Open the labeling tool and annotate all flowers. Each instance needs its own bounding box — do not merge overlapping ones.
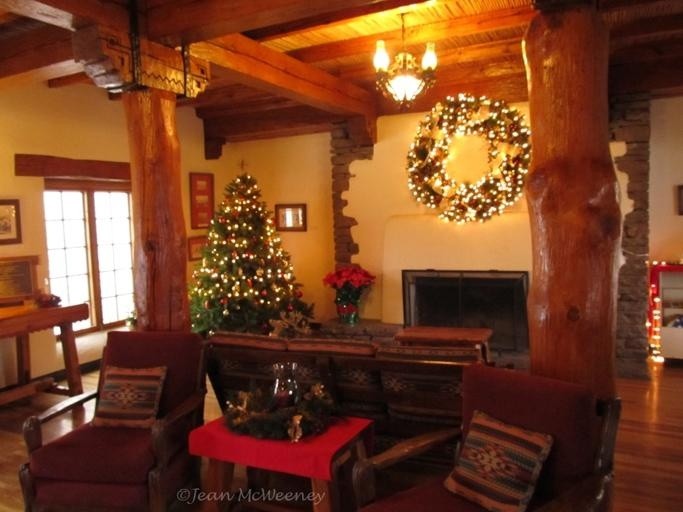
[323,262,376,304]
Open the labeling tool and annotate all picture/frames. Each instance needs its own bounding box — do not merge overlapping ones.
[190,236,207,260]
[0,198,23,244]
[0,255,38,303]
[275,204,306,232]
[190,173,214,231]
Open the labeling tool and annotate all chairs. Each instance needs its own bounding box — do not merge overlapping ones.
[287,331,383,428]
[208,328,288,414]
[376,344,491,424]
[364,364,621,511]
[19,330,205,511]
[372,12,437,109]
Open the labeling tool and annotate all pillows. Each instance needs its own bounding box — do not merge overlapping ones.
[447,410,553,512]
[93,366,168,429]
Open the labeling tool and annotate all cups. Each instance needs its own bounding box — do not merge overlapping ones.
[270,361,300,408]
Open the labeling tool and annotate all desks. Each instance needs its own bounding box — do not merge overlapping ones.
[310,319,404,341]
[1,304,89,408]
[187,416,377,511]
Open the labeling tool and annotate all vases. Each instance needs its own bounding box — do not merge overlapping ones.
[340,302,359,321]
